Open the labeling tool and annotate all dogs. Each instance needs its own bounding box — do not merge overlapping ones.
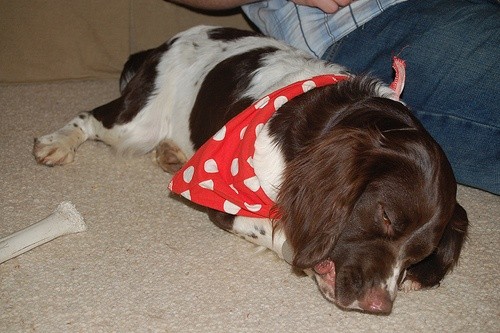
[32,23,469,316]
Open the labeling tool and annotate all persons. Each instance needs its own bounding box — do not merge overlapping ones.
[170,0,500,196]
